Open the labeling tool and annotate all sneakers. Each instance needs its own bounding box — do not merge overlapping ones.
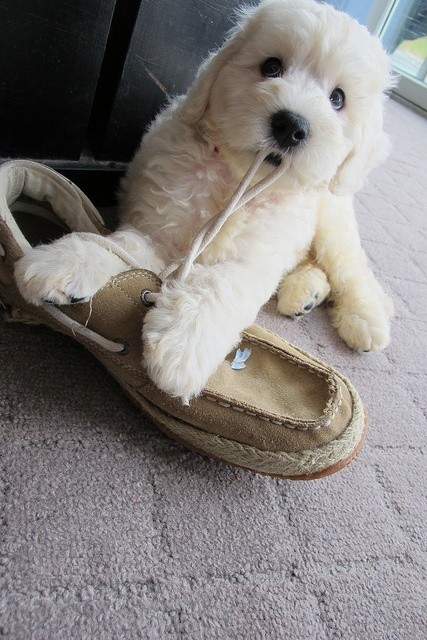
[1,159,368,480]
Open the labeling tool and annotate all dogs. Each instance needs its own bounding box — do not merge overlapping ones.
[12,0,396,398]
